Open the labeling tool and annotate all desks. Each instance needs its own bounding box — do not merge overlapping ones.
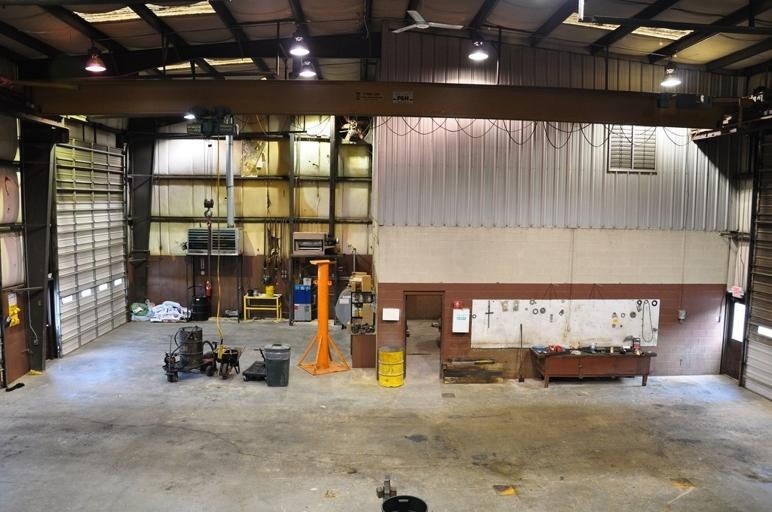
[529,345,656,387]
[289,256,339,327]
[242,291,282,320]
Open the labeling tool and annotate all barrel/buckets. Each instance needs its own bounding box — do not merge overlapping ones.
[180,326,202,367]
[191,295,210,321]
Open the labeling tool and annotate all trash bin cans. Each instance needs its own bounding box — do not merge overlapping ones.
[264,343,291,385]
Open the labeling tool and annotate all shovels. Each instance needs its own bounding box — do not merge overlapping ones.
[520,324,523,382]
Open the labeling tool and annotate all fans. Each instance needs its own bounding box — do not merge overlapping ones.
[390,9,464,36]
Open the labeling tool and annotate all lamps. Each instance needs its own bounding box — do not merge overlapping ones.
[83,34,107,72]
[658,54,683,89]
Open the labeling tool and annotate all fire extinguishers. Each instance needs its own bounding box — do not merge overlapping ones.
[205,281,212,298]
